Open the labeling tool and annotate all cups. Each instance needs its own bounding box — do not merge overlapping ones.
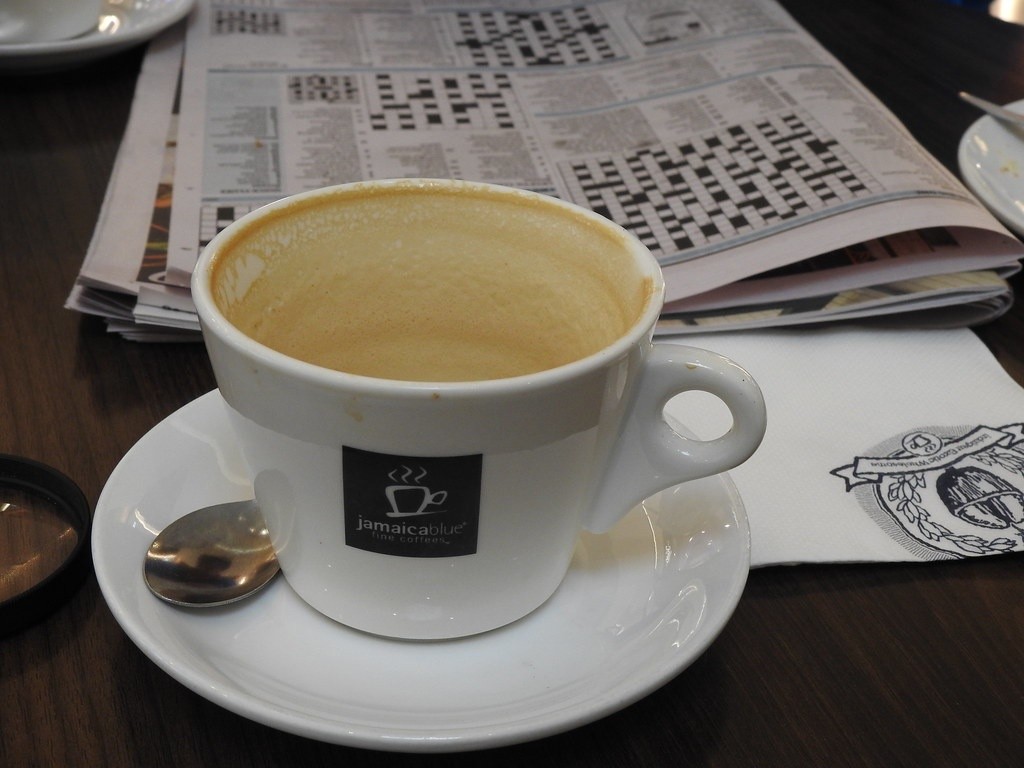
[191,179,766,643]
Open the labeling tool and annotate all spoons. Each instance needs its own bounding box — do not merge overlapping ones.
[144,499,277,610]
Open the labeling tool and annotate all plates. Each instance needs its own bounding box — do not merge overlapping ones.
[90,386,753,752]
[956,96,1024,236]
[0,0,195,56]
